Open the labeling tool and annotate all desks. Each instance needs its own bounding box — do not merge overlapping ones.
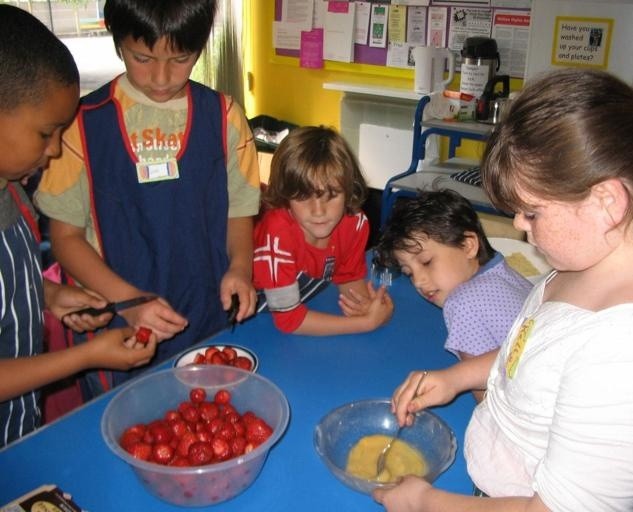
[1,238,559,512]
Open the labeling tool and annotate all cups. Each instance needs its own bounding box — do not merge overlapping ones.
[486,97,512,126]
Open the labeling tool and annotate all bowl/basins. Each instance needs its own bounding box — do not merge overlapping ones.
[170,342,260,398]
[98,364,290,510]
[315,395,459,499]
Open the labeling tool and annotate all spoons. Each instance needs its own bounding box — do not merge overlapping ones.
[372,371,428,476]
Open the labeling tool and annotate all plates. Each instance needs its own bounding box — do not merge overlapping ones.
[484,234,552,287]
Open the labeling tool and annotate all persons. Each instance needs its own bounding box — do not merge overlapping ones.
[371,175,538,406]
[371,66,632,511]
[0,0,158,449]
[33,0,263,408]
[252,125,394,338]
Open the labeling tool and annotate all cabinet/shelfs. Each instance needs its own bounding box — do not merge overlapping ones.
[377,90,530,234]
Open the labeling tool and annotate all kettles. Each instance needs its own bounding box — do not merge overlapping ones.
[409,44,454,96]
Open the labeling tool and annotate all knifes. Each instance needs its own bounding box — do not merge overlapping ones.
[59,292,159,324]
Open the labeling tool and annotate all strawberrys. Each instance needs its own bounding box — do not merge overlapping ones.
[179,345,252,386]
[135,326,152,344]
[117,388,274,508]
